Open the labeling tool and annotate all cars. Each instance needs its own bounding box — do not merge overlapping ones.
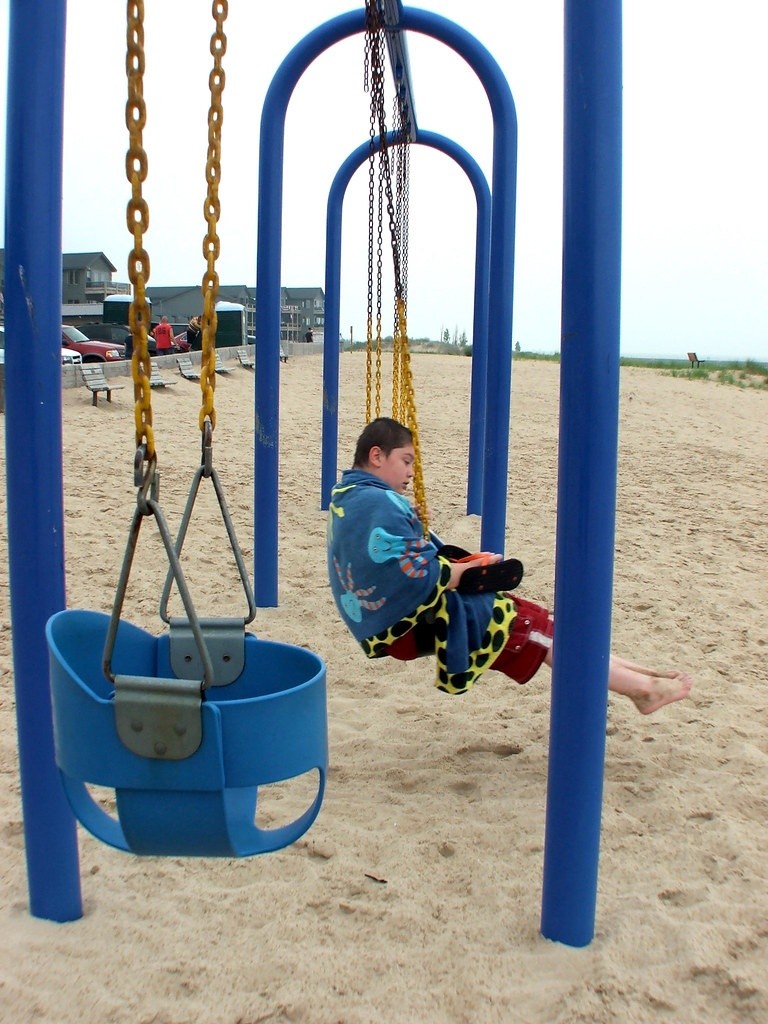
[0,326,84,366]
[61,324,126,363]
[171,331,192,353]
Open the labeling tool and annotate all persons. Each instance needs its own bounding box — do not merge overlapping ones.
[327,417,693,715]
[187,314,202,348]
[305,328,313,343]
[124,329,133,360]
[150,316,180,355]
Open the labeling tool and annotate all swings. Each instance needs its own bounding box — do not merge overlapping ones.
[364,0,433,663]
[44,0,329,860]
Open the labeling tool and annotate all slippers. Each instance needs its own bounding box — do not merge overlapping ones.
[459,556,523,593]
[435,545,496,563]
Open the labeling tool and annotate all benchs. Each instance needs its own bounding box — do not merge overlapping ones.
[280,345,294,363]
[140,362,178,389]
[79,364,125,407]
[214,354,237,374]
[688,352,705,368]
[236,349,254,368]
[176,358,201,379]
